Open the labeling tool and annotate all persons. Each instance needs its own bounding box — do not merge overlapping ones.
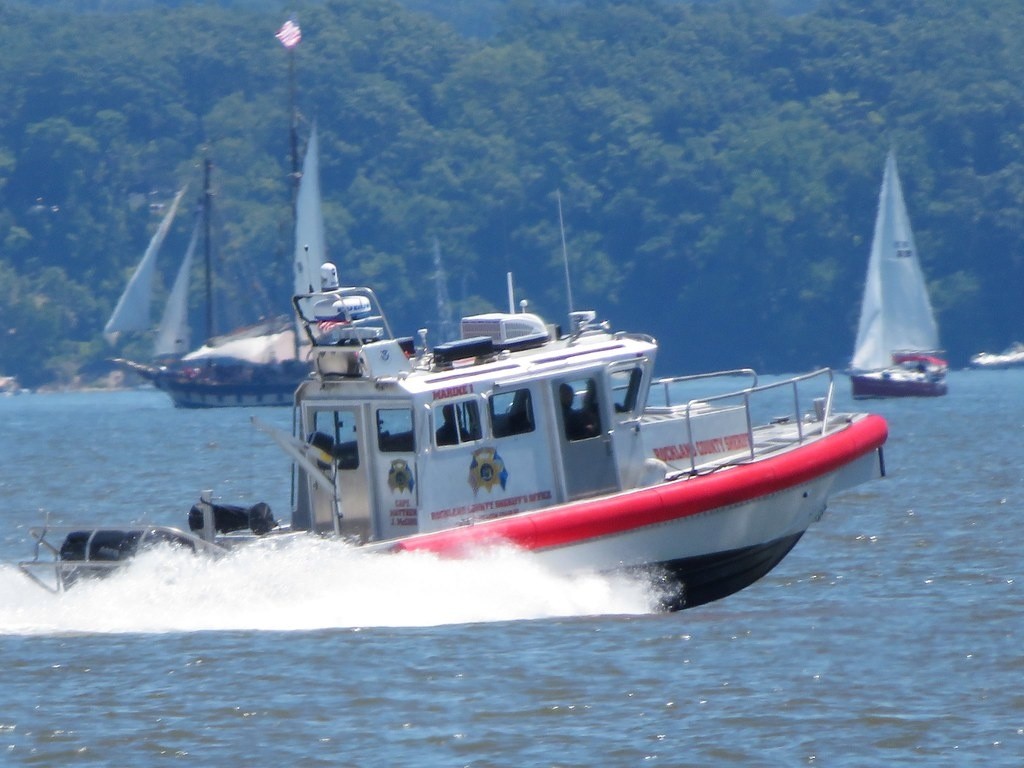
[560,383,598,440]
[916,361,925,371]
[435,404,470,446]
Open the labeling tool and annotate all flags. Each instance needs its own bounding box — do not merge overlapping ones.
[275,11,302,50]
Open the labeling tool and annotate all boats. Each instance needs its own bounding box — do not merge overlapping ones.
[2,187,886,622]
[968,341,1024,373]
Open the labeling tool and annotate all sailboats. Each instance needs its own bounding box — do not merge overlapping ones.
[99,16,417,410]
[844,155,948,402]
